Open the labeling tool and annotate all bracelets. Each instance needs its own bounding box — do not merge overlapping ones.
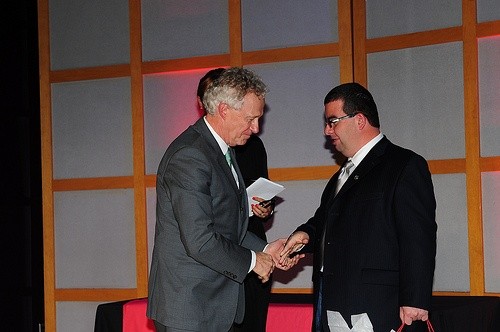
[270,209,273,216]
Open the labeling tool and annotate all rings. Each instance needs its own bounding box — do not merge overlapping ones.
[259,212,263,215]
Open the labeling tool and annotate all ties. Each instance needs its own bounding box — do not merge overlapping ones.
[334,159,354,192]
[225,150,239,181]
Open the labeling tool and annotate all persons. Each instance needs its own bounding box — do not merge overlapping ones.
[197,67,273,332]
[279,82,438,332]
[146,67,305,332]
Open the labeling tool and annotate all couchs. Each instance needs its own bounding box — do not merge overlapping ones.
[93,293,500,332]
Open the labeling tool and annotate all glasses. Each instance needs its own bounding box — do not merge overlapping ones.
[326,112,356,126]
